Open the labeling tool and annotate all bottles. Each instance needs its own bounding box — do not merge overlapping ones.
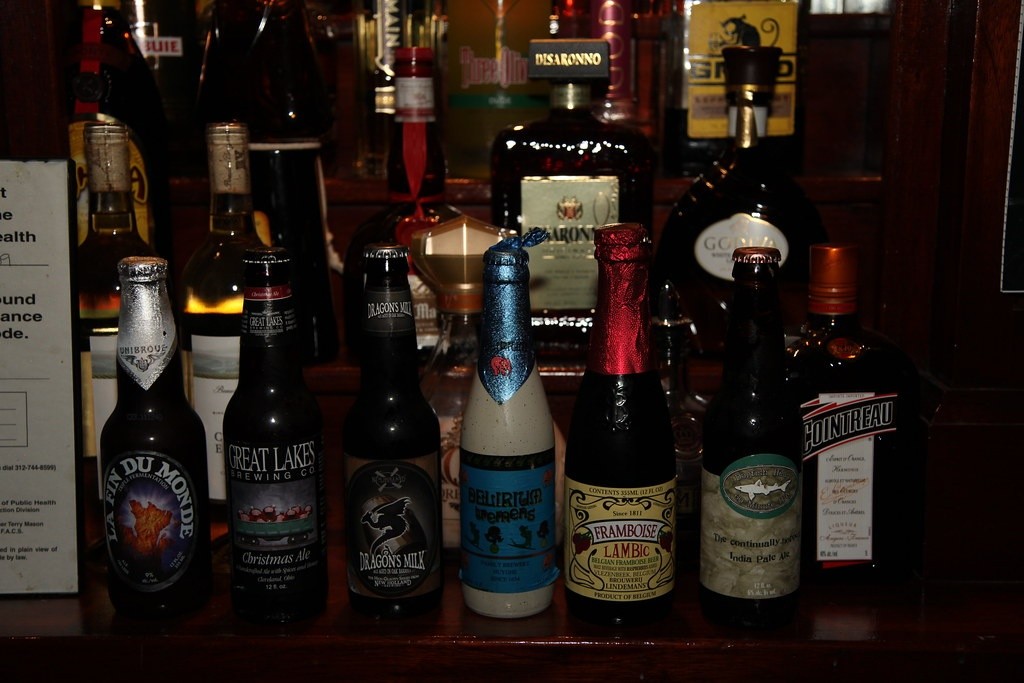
[701,248,809,624]
[222,243,326,632]
[71,121,159,549]
[656,43,827,358]
[342,247,443,613]
[101,255,212,622]
[636,281,709,575]
[414,217,516,552]
[248,141,334,366]
[490,41,654,356]
[341,44,467,361]
[561,225,682,623]
[178,121,257,523]
[461,248,557,618]
[787,242,918,588]
[63,0,809,186]
